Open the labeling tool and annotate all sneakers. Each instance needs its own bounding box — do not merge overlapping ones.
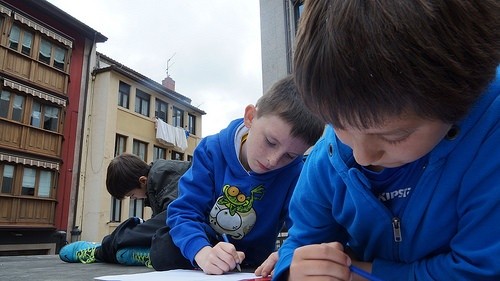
[116,246,154,269]
[59,241,104,263]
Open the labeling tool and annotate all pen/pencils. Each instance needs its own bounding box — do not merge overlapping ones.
[221,233,243,273]
[347,264,383,281]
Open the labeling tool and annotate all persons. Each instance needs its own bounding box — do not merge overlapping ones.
[59,74,325,278]
[106,153,192,218]
[273,1,500,281]
